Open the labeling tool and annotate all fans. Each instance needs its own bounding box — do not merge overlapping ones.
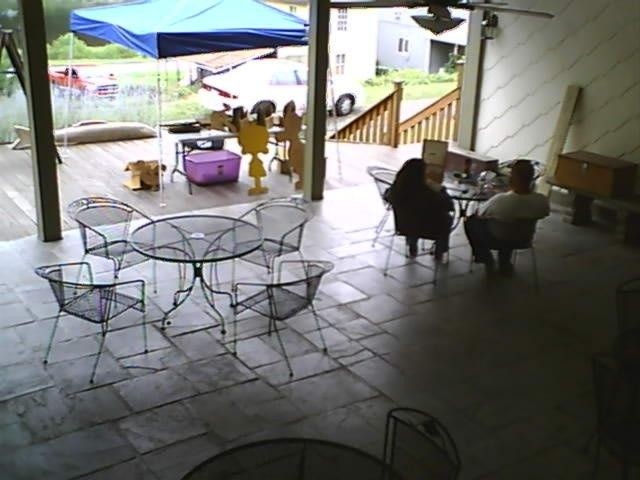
[324,0,558,24]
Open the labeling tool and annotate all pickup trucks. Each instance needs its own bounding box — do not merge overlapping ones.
[49,63,119,101]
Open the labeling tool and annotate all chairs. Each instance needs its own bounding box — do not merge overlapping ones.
[227,257,334,382]
[365,158,556,289]
[34,261,153,387]
[578,275,639,480]
[375,402,464,480]
[63,194,159,302]
[224,194,319,291]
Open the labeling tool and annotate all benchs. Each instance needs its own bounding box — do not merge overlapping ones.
[545,173,615,231]
[590,192,639,243]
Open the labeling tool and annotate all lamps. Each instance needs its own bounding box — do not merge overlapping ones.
[479,11,503,42]
[408,6,465,35]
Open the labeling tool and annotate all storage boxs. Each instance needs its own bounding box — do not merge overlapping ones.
[555,149,638,202]
[183,145,243,187]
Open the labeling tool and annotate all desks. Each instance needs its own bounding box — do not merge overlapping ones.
[165,122,307,197]
[122,215,267,336]
[174,433,407,478]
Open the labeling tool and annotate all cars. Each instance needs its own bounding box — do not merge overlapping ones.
[198,57,365,120]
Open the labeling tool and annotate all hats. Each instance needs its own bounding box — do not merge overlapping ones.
[507,160,535,183]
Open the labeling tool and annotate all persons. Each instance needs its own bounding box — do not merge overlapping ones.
[383,156,453,255]
[463,161,550,270]
